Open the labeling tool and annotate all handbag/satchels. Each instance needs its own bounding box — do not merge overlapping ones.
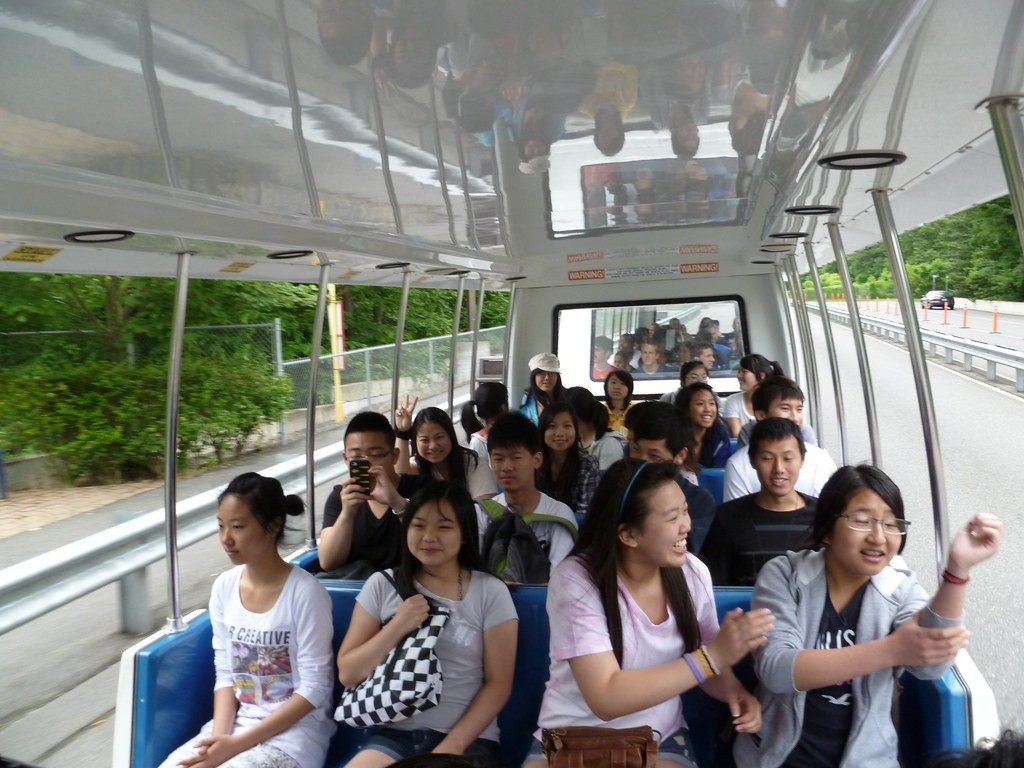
[334,571,451,729]
[538,726,661,768]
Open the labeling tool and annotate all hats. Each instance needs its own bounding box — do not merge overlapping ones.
[528,352,561,374]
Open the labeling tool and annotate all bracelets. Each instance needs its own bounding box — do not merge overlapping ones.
[395,425,412,440]
[697,645,720,677]
[391,498,410,517]
[683,654,704,686]
[943,571,968,584]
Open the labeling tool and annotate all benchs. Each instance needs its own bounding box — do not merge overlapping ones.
[113,438,999,767]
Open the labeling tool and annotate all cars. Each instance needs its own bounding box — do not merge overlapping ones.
[921,291,954,310]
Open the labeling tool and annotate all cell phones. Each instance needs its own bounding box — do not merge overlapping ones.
[350,460,370,497]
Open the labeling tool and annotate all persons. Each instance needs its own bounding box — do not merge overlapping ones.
[395,394,498,502]
[319,411,428,578]
[701,417,818,586]
[521,352,697,524]
[461,383,508,454]
[658,354,839,502]
[633,401,715,553]
[160,473,336,768]
[520,458,774,768]
[731,463,1001,768]
[474,412,579,585]
[316,0,793,224]
[336,479,519,768]
[594,318,742,378]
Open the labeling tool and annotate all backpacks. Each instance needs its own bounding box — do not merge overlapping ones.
[471,497,578,586]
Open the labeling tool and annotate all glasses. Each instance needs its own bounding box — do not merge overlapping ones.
[343,451,392,466]
[833,512,911,535]
